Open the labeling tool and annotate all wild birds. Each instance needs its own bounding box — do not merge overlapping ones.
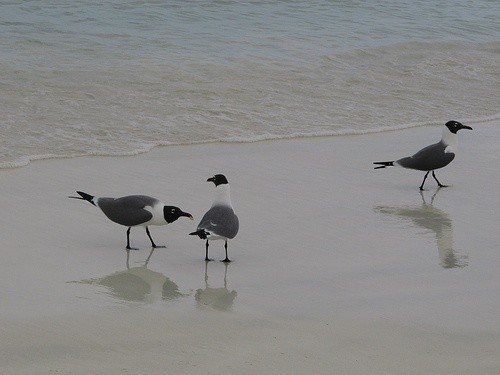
[188,173,239,262]
[65,247,193,311]
[373,120,473,190]
[67,188,194,251]
[373,187,470,268]
[194,261,238,312]
